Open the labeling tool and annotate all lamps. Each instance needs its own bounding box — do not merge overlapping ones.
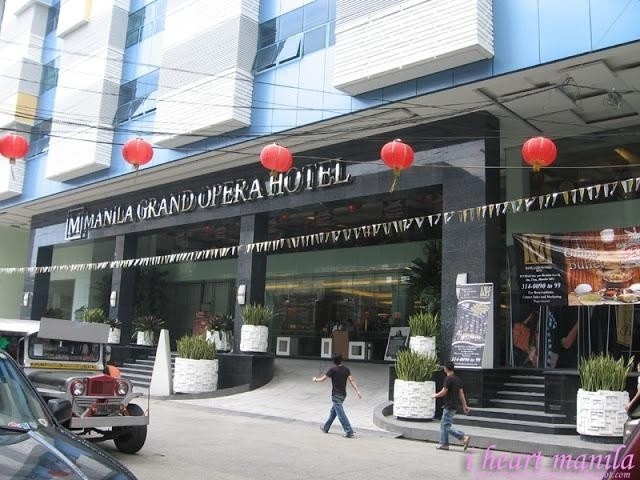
[456,273,469,301]
[23,292,30,307]
[110,290,117,308]
[237,283,247,306]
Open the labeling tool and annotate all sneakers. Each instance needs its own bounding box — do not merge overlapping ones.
[320,426,354,437]
[436,436,471,451]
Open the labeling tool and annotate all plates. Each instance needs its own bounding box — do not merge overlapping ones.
[578,293,604,306]
[598,287,621,299]
[602,270,633,283]
[616,293,640,305]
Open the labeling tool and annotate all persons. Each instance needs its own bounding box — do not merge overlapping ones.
[430,360,470,451]
[330,319,344,336]
[344,319,356,341]
[312,351,362,440]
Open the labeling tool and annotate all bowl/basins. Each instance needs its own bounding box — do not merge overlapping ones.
[574,288,592,297]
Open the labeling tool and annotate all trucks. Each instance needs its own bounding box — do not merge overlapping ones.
[0,316,148,454]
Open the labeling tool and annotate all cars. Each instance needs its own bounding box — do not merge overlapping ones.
[0,349,140,480]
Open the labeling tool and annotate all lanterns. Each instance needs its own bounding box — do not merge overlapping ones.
[122,137,153,168]
[521,136,558,170]
[380,136,413,177]
[260,143,292,177]
[0,132,29,164]
